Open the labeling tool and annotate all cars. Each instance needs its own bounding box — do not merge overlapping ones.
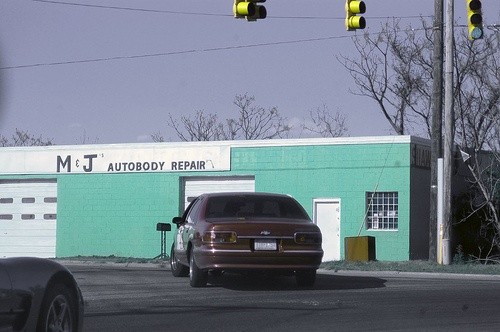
[170,192,324,287]
[0,258,84,331]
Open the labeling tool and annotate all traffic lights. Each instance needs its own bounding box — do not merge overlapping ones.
[466,0,483,40]
[233,0,269,23]
[345,0,366,31]
